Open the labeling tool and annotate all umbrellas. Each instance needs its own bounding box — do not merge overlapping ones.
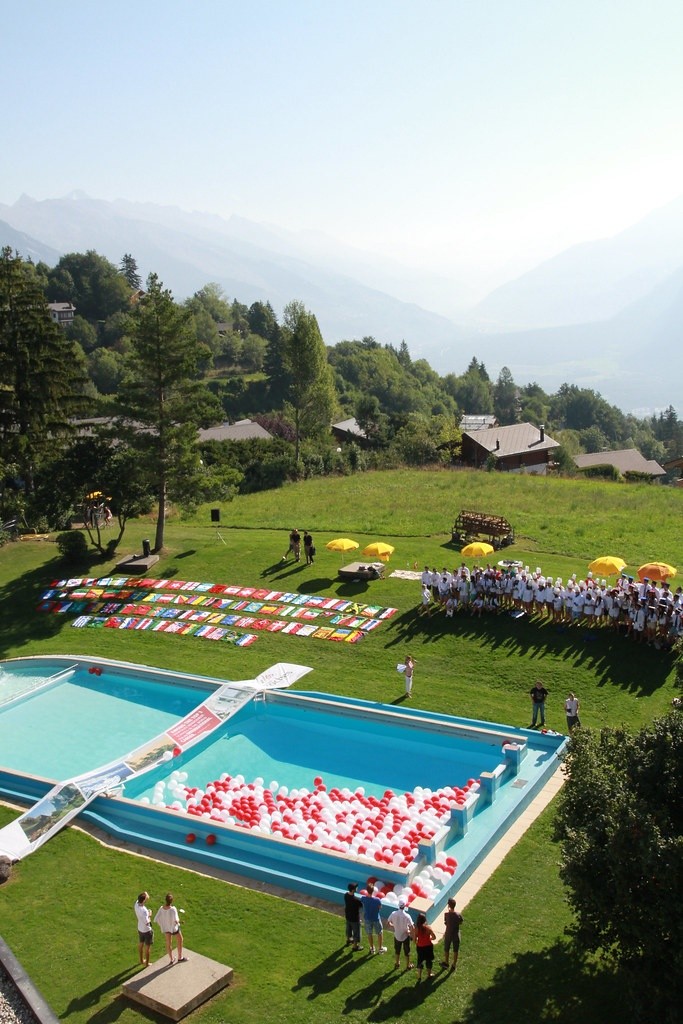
[588,556,627,585]
[461,542,494,567]
[637,562,677,584]
[326,538,395,563]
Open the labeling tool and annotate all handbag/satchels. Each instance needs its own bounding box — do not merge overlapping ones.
[310,548,317,555]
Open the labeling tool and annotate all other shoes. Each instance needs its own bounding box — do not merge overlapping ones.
[407,963,415,970]
[379,946,387,954]
[352,945,364,951]
[346,939,354,944]
[439,961,449,968]
[395,960,400,968]
[427,974,435,978]
[178,958,188,963]
[451,963,457,970]
[169,957,177,964]
[370,946,376,954]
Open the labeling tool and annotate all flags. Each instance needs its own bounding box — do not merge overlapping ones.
[36,578,398,647]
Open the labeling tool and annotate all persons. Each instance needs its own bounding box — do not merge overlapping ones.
[153,893,190,964]
[361,883,387,954]
[529,682,548,727]
[85,502,113,528]
[421,561,683,650]
[404,656,415,698]
[344,883,364,951]
[440,898,463,971]
[413,913,436,980]
[134,891,155,967]
[387,901,414,970]
[283,529,315,565]
[564,693,581,731]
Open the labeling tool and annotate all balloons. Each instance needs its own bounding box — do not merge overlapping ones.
[142,740,523,907]
[88,667,101,676]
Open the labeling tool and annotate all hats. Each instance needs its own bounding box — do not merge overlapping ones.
[507,561,606,596]
[347,883,359,891]
[607,574,683,613]
[399,899,407,908]
